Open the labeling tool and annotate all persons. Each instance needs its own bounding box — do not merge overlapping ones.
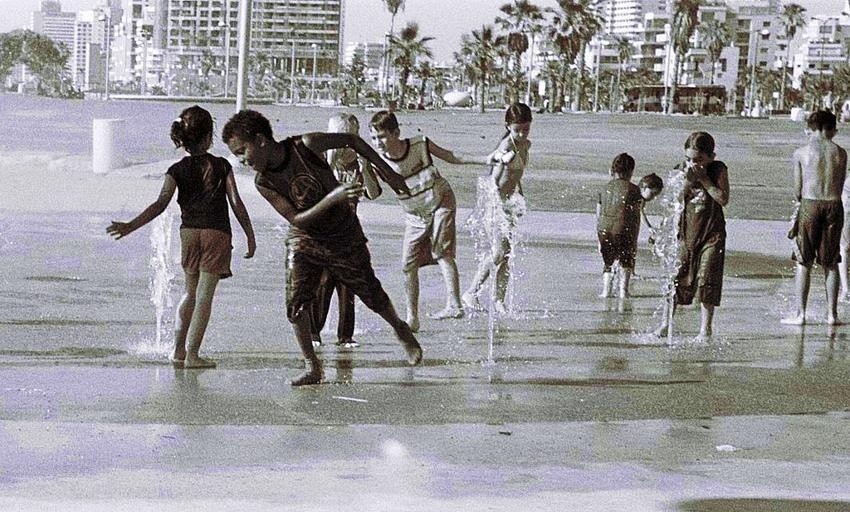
[780,110,847,325]
[221,108,424,389]
[595,152,640,304]
[649,131,731,343]
[610,170,664,279]
[839,176,850,303]
[368,113,501,334]
[103,105,256,368]
[310,112,384,352]
[460,101,534,320]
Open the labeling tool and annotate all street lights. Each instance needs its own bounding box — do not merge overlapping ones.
[136,28,148,95]
[748,28,770,115]
[218,21,231,98]
[811,15,840,111]
[310,43,317,105]
[97,7,111,101]
